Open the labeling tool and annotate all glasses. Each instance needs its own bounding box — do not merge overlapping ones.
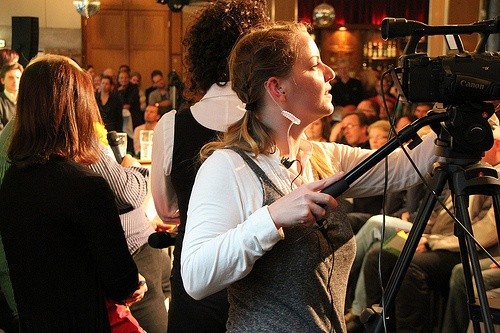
[368,134,388,139]
[346,123,360,129]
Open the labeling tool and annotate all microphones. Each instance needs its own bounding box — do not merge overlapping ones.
[281,109,301,125]
[106,131,123,164]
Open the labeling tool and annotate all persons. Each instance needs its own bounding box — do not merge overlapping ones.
[180,21,446,333]
[304,62,500,333]
[151,0,307,333]
[0,49,173,332]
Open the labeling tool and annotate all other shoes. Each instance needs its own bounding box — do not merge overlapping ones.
[344,308,365,333]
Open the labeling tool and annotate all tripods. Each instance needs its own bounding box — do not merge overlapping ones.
[314,103,500,333]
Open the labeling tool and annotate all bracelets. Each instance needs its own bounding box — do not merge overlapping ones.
[424,243,432,251]
